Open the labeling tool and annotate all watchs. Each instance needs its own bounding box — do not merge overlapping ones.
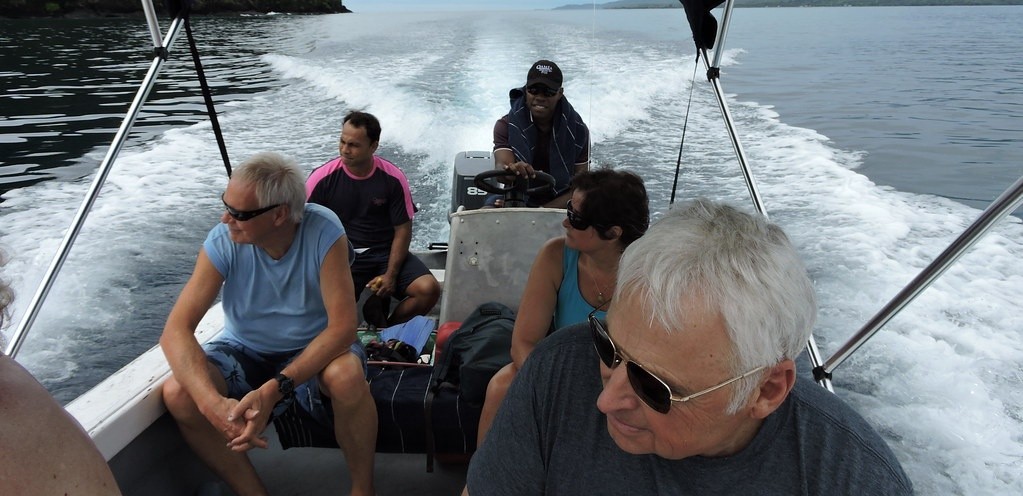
[275,374,294,395]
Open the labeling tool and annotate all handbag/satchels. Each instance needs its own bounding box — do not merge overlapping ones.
[432,301,515,403]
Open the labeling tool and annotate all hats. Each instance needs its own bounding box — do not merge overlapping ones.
[527,60,563,91]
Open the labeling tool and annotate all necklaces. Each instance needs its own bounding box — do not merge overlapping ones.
[591,274,610,303]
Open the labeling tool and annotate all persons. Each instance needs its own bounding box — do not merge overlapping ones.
[463,197,908,495]
[482,60,592,208]
[160,153,378,495]
[304,111,441,324]
[0,242,121,496]
[477,165,652,450]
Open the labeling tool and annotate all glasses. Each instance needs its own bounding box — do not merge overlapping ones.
[222,192,280,221]
[566,199,625,232]
[587,296,767,415]
[526,84,561,97]
